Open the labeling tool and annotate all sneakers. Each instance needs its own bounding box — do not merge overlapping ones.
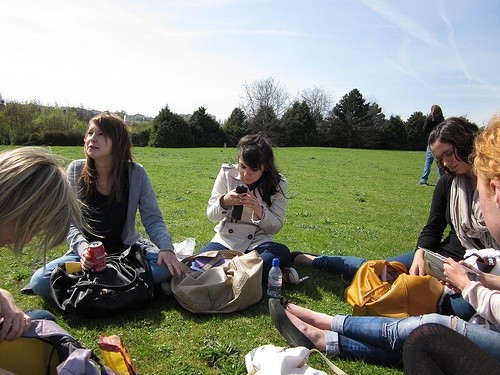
[282,267,299,286]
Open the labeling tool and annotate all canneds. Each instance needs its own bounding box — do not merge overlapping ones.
[88,241,107,272]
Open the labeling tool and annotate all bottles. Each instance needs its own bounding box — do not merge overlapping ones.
[267,258,283,298]
[467,309,490,332]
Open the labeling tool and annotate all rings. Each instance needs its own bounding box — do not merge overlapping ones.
[167,264,171,266]
[240,200,242,203]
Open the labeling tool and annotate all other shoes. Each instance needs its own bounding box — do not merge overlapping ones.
[160,282,171,296]
[277,296,289,309]
[290,251,321,266]
[269,298,316,351]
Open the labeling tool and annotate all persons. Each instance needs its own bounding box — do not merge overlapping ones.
[418,105,445,185]
[200,134,298,287]
[0,146,104,375]
[269,117,500,375]
[30,111,183,325]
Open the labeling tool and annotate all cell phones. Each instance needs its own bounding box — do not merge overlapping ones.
[236,186,247,196]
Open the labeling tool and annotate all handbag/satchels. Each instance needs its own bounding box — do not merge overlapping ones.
[172,251,265,315]
[344,259,444,319]
[50,244,157,323]
[0,319,118,375]
[243,343,347,375]
